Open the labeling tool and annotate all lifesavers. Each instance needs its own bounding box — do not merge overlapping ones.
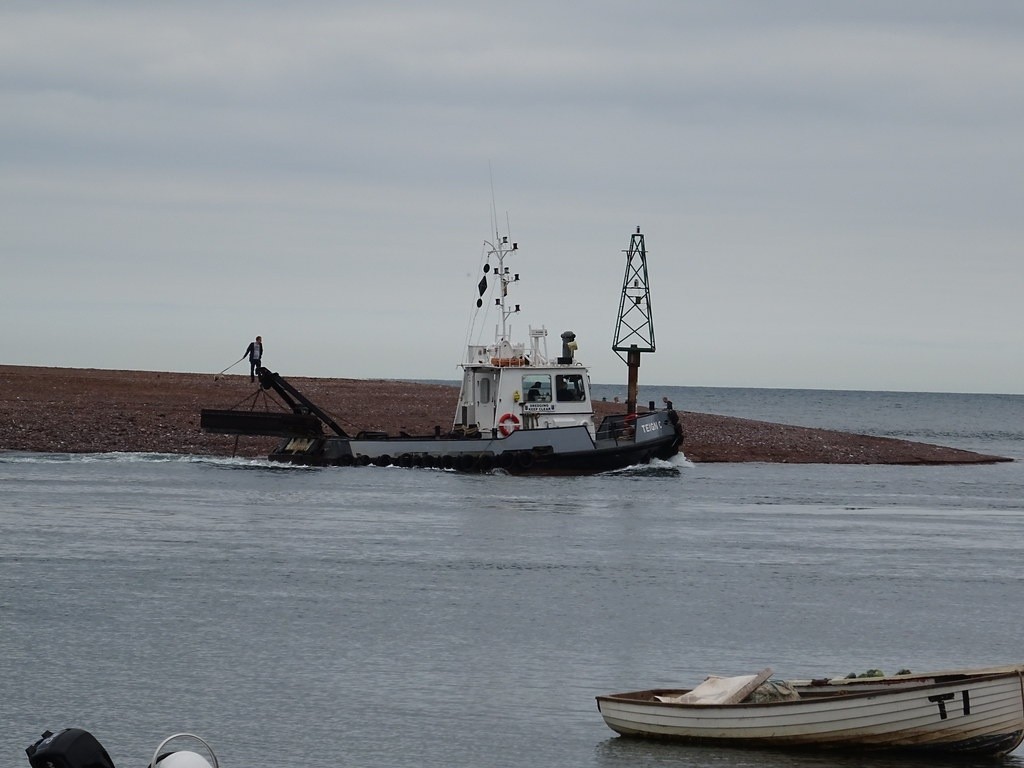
[624,414,639,434]
[499,414,519,436]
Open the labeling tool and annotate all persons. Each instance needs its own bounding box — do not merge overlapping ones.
[528,382,541,401]
[243,336,263,383]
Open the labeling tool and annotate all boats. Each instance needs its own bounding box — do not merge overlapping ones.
[347,164,682,474]
[594,666,1023,765]
